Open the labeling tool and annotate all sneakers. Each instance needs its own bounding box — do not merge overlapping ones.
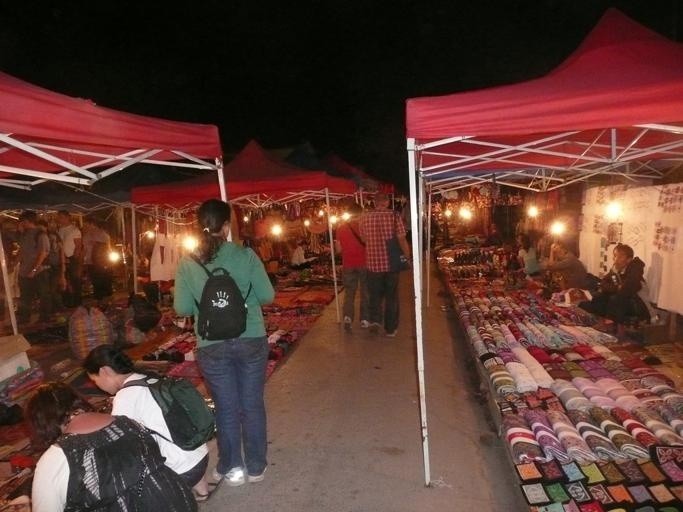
[344,317,398,337]
[211,467,267,487]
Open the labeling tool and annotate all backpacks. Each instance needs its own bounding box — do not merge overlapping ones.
[119,370,215,451]
[190,253,252,341]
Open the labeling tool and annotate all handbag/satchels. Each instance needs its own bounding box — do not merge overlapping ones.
[387,237,410,273]
[68,307,115,361]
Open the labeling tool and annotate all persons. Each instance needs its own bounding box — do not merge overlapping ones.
[86,343,216,502]
[288,240,319,271]
[171,198,275,487]
[429,206,590,291]
[588,242,655,324]
[4,208,115,310]
[398,192,412,255]
[19,377,198,512]
[333,201,372,334]
[357,193,412,339]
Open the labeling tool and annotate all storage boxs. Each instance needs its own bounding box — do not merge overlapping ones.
[0,334,33,382]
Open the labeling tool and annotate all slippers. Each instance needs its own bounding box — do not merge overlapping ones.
[196,477,224,504]
